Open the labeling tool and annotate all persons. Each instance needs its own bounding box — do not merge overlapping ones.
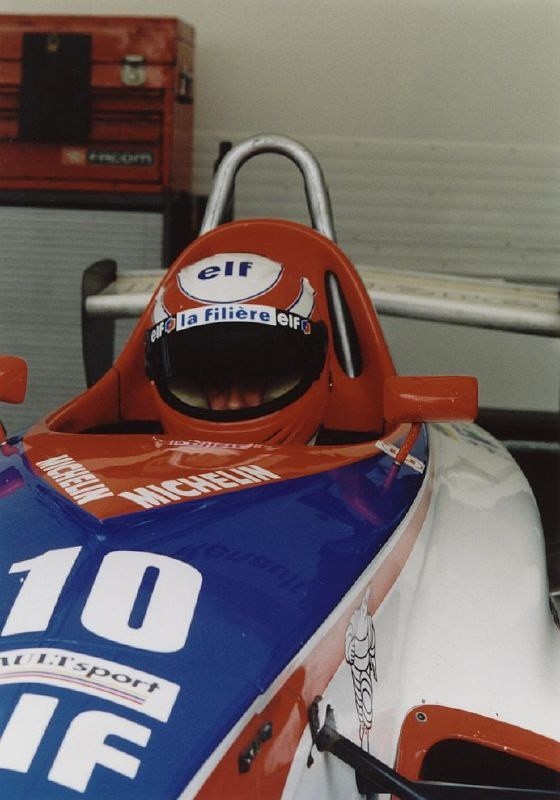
[135,226,362,448]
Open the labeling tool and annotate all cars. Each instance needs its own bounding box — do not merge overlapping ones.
[0,130,560,800]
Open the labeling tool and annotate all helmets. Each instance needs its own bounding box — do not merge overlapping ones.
[147,253,333,446]
